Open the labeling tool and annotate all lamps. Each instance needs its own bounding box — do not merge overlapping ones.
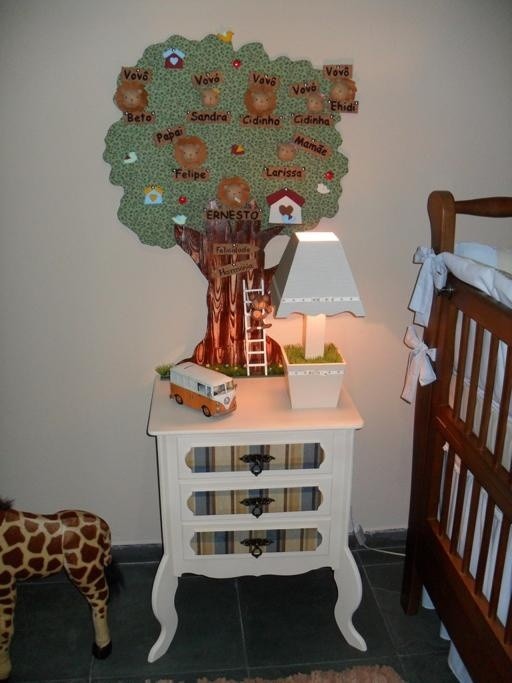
[268,231,364,411]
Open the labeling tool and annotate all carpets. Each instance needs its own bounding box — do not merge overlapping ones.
[143,664,404,682]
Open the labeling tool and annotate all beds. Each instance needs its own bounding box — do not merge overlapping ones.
[400,189,512,682]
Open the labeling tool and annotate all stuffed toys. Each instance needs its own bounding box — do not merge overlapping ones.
[0,479,113,680]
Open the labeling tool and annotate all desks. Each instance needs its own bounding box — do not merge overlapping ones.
[147,370,369,662]
